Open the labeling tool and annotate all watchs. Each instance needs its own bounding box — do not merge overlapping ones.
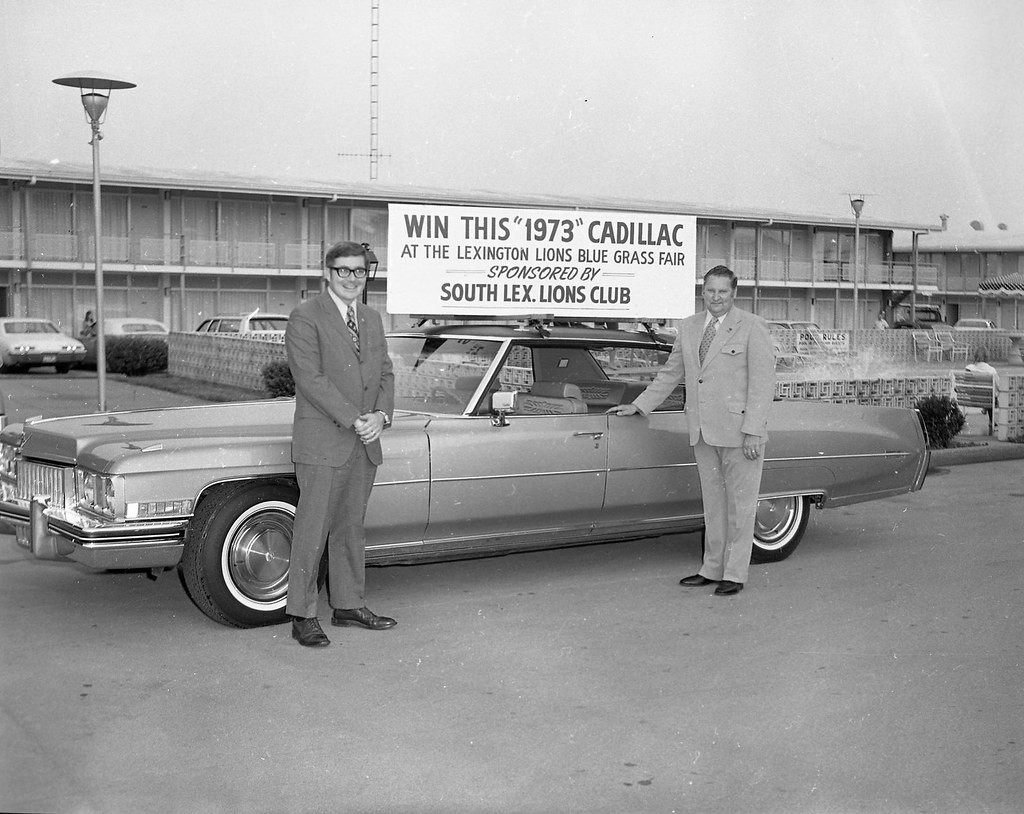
[379,411,390,425]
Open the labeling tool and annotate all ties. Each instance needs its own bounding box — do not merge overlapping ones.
[699,316,719,367]
[345,306,360,356]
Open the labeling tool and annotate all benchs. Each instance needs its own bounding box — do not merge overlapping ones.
[566,379,686,413]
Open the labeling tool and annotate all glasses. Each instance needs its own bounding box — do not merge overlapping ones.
[330,267,367,278]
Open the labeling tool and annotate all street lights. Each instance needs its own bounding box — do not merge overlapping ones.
[52,76,138,414]
[849,194,864,356]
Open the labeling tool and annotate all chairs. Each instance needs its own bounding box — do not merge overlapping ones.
[793,344,818,366]
[446,376,588,414]
[772,336,804,371]
[911,331,943,362]
[935,332,969,363]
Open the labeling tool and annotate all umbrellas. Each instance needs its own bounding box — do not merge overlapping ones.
[979,273,1024,328]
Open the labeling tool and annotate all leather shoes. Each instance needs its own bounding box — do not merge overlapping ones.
[292,616,330,646]
[714,580,743,595]
[679,574,713,586]
[331,607,397,630]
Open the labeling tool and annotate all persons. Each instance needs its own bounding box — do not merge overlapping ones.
[285,241,398,647]
[83,311,96,337]
[872,314,890,329]
[607,265,774,596]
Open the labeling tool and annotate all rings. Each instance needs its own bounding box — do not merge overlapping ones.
[751,451,755,452]
[371,430,376,435]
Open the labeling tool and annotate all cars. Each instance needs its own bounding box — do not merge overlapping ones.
[955,318,998,330]
[8,314,934,629]
[70,316,169,370]
[0,317,87,375]
[195,313,289,333]
